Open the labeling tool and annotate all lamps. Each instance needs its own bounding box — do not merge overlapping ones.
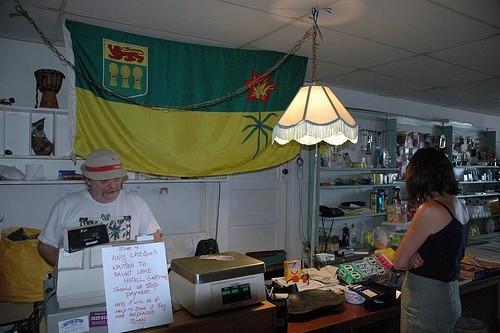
[271,11,360,146]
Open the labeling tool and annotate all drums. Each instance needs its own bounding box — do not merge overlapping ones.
[365,226,389,250]
[34,68,65,109]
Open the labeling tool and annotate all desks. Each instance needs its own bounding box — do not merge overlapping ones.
[125,241,500,333]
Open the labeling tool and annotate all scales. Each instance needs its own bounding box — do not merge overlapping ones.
[167,251,267,317]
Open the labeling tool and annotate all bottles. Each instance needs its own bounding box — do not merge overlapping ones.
[463,168,500,182]
[342,223,350,247]
[350,223,357,246]
[360,221,367,244]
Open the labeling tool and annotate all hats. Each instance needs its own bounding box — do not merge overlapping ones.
[81,150,127,180]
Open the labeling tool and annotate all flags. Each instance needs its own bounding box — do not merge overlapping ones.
[61,19,309,177]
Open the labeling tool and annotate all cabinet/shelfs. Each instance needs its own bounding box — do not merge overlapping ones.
[308,115,500,270]
[0,105,85,184]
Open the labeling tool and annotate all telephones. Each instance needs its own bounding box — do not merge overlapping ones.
[319,205,345,217]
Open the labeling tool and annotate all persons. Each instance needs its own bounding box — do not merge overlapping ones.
[394,147,471,333]
[37,150,163,268]
